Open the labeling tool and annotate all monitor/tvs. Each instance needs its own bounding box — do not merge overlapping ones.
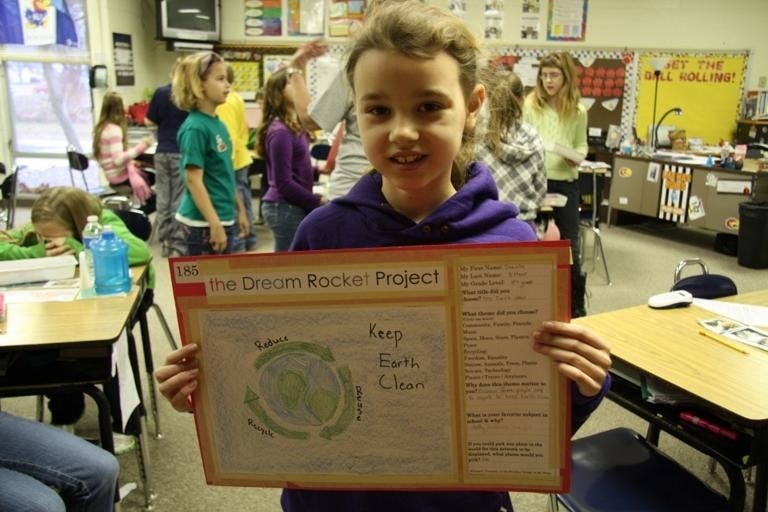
[155,0,222,44]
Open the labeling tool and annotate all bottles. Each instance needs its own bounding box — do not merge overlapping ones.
[619,139,638,157]
[82,214,104,289]
[79,252,91,289]
[706,154,713,168]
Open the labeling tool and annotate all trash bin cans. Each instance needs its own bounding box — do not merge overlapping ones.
[738,202,768,269]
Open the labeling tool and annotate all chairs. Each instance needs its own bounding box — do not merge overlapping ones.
[580,164,614,282]
[116,207,185,402]
[656,257,740,299]
[310,140,336,164]
[64,144,157,200]
[549,427,738,507]
[1,164,19,231]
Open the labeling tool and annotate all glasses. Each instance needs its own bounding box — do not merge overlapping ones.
[539,72,563,81]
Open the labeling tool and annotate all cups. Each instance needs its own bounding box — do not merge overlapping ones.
[0,304,7,335]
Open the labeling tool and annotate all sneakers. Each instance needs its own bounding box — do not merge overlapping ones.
[112,431,141,455]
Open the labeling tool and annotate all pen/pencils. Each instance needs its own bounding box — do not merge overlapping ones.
[698,330,749,355]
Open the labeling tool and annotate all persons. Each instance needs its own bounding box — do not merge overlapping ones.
[152,0,612,512]
[90,35,373,255]
[0,184,157,455]
[467,52,589,319]
[0,409,121,511]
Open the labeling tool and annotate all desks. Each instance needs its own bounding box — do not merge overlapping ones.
[574,287,768,507]
[607,153,768,235]
[1,262,156,506]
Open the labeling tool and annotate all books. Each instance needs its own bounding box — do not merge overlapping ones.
[243,0,282,37]
